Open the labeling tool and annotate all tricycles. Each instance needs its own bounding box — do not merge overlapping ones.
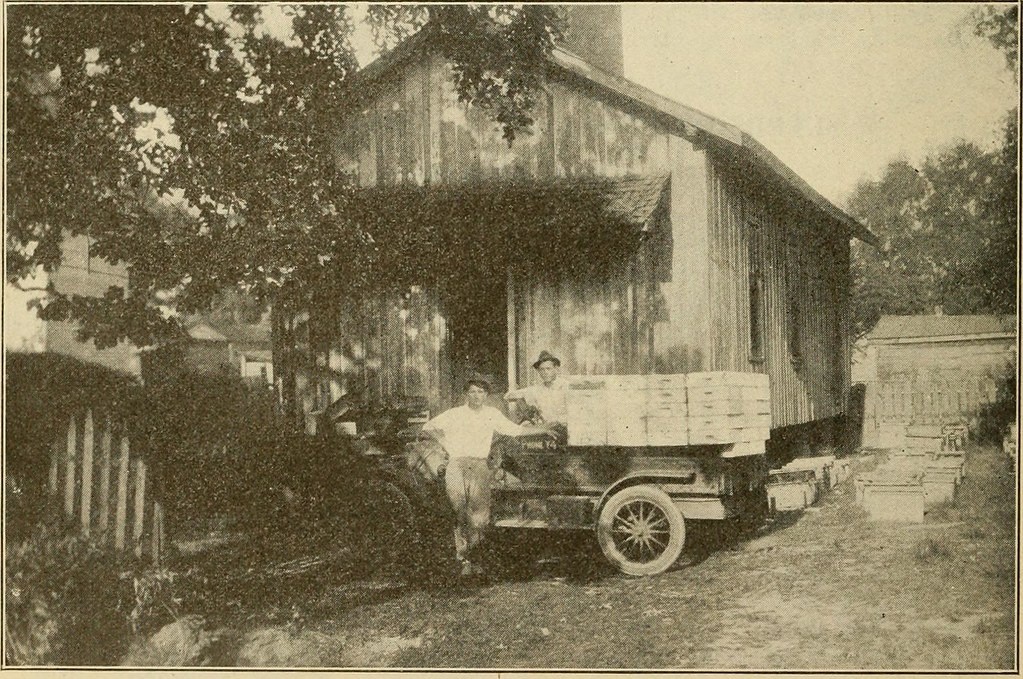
[347,391,765,577]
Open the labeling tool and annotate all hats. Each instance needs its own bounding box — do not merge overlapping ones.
[532,349,561,369]
[463,375,492,395]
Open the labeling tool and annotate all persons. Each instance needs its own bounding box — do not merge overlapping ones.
[502,352,567,450]
[423,377,560,576]
